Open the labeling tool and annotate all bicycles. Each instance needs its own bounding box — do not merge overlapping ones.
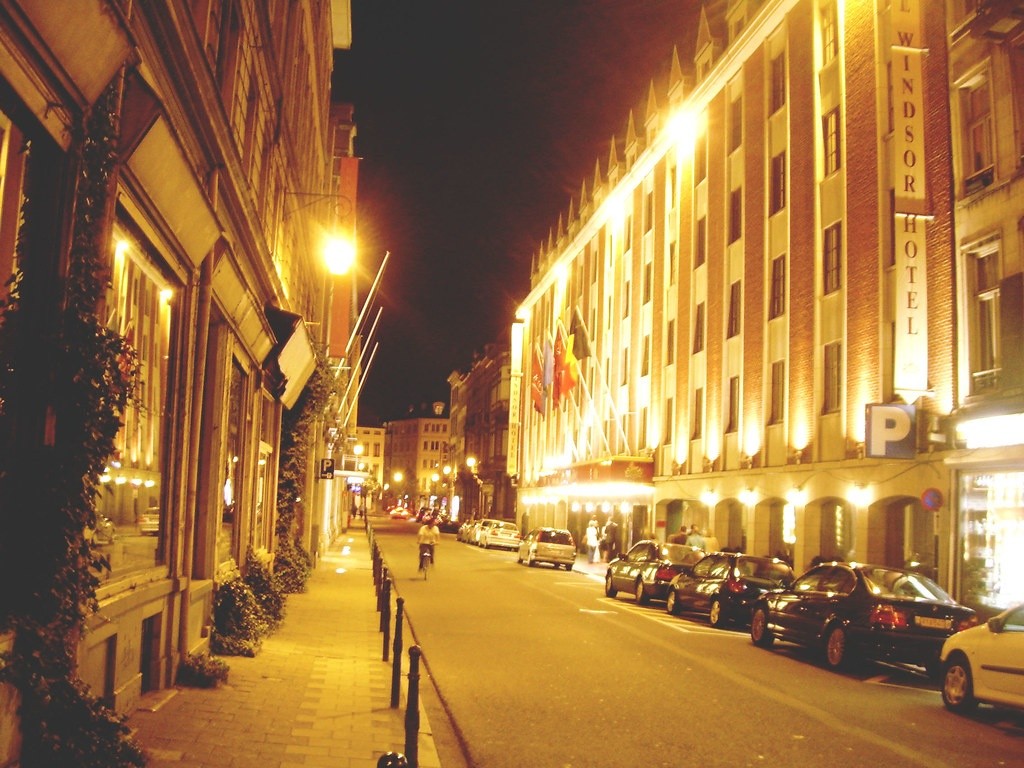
[423,551,430,580]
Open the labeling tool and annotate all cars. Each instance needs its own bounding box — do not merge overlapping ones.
[382,500,519,551]
[665,552,795,627]
[940,605,1023,716]
[749,562,980,673]
[517,527,578,570]
[605,540,704,603]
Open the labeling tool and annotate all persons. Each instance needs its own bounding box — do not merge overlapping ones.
[359,502,364,518]
[416,519,439,570]
[352,504,358,518]
[669,524,719,551]
[586,515,618,564]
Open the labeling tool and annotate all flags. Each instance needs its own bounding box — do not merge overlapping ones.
[531,310,591,422]
